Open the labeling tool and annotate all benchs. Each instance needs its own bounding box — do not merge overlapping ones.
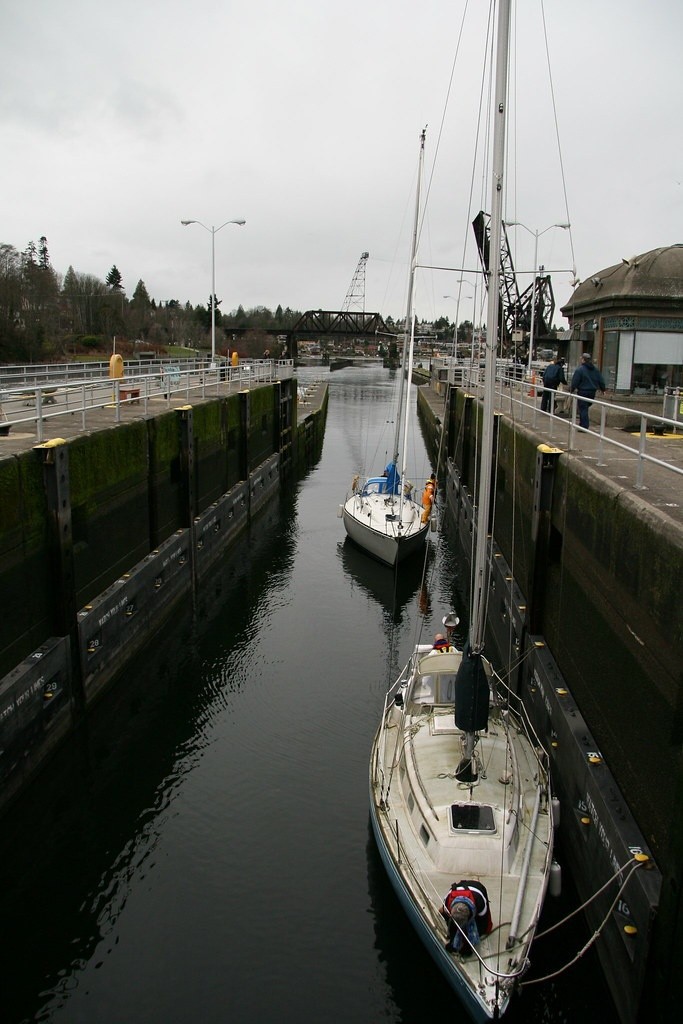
[22,387,57,407]
[119,388,140,405]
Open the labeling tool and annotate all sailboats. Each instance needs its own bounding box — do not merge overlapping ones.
[367,0,580,1024]
[338,118,435,567]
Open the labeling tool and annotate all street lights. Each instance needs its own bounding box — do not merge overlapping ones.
[505,219,571,378]
[180,218,250,371]
[442,295,473,364]
[459,278,489,368]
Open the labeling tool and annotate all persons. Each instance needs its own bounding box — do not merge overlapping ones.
[421,474,437,524]
[422,634,458,690]
[403,481,413,500]
[443,880,492,939]
[541,360,567,415]
[568,353,606,432]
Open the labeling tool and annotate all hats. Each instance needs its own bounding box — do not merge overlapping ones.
[580,353,591,358]
[556,359,565,364]
